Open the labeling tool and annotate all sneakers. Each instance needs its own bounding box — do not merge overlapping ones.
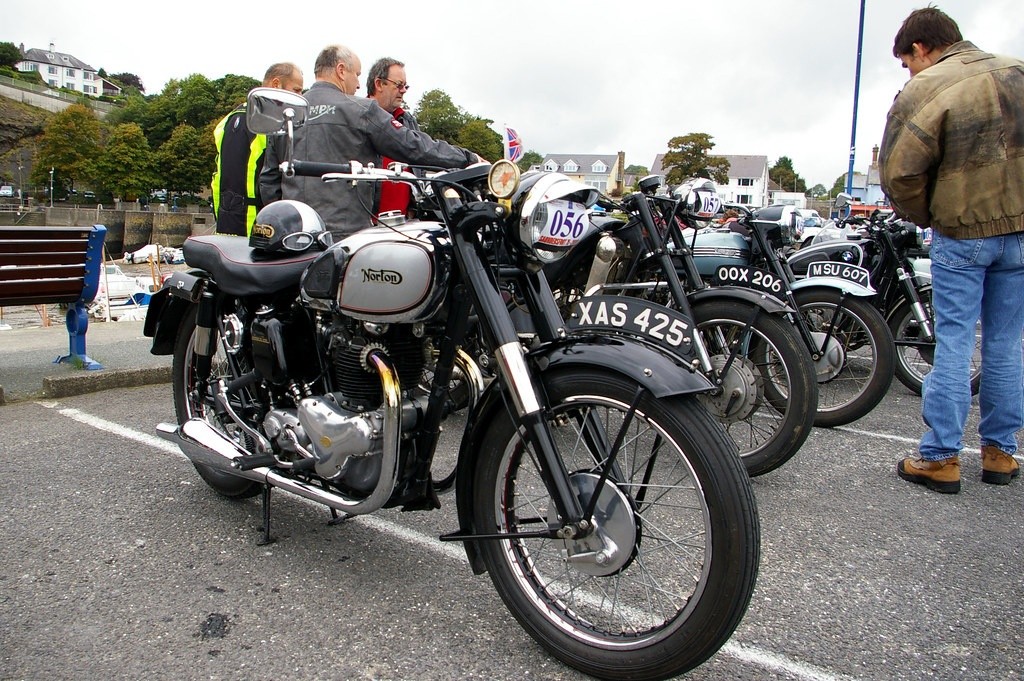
[981,445,1019,484]
[897,456,961,493]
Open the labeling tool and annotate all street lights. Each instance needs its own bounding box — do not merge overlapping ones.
[49,170,54,208]
[18,166,25,205]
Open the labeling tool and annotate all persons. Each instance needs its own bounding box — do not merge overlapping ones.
[258,45,490,242]
[878,9,1024,495]
[365,57,426,225]
[211,62,303,239]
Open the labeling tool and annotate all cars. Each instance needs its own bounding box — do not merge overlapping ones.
[84,190,95,198]
[153,193,168,203]
[66,189,78,201]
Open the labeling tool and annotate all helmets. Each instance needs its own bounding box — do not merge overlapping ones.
[249,199,337,252]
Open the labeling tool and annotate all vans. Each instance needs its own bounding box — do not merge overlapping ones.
[0,186,15,197]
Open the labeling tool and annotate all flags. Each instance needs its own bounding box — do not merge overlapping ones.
[504,128,524,163]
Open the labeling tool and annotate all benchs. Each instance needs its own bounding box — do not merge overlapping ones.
[0,225,107,370]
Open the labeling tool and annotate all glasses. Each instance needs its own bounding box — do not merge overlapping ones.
[279,231,334,251]
[380,77,409,90]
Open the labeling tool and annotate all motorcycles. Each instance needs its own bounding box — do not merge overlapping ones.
[126,159,991,681]
